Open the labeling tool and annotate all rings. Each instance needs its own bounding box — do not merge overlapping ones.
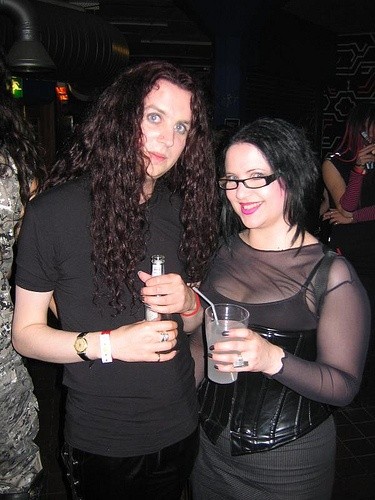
[330,211,335,213]
[160,333,168,342]
[158,353,160,362]
[233,353,248,368]
[329,215,332,218]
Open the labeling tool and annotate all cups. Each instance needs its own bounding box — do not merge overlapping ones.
[366,160,374,169]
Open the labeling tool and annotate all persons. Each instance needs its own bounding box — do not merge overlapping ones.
[12,59,221,500]
[189,116,372,500]
[0,62,47,500]
[298,102,375,389]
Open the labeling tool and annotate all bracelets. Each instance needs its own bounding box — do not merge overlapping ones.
[352,169,363,174]
[100,331,113,363]
[355,163,365,167]
[181,293,200,317]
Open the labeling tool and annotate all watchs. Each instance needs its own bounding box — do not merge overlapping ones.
[75,331,95,368]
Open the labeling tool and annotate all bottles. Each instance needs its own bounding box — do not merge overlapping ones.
[360,130,375,146]
[145,255,173,359]
[205,303,249,383]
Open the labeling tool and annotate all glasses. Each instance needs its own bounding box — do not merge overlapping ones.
[218,172,283,190]
